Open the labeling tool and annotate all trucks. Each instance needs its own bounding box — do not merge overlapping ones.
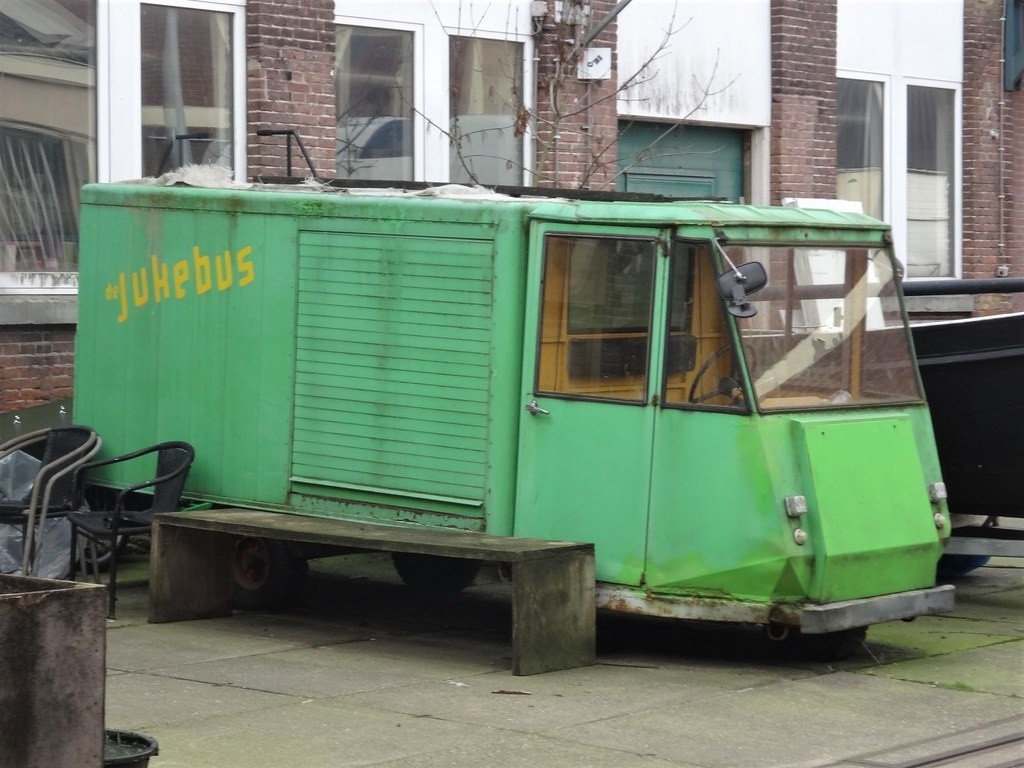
[68,176,955,662]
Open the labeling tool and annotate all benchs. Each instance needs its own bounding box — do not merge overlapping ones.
[148,508,597,676]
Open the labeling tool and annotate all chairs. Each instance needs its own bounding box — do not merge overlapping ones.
[1,425,102,577]
[67,441,196,620]
